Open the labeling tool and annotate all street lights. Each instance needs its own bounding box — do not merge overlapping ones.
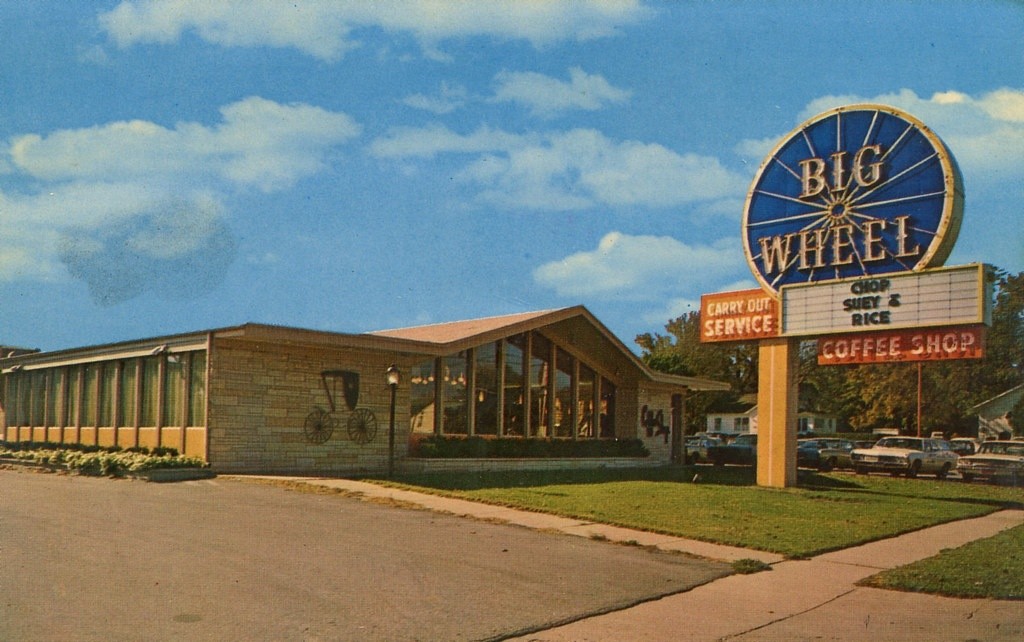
[386,364,400,475]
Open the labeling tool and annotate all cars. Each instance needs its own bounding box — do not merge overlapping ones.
[957,440,1023,482]
[848,437,959,479]
[686,432,718,465]
[950,438,989,455]
[796,441,853,471]
[706,432,757,467]
